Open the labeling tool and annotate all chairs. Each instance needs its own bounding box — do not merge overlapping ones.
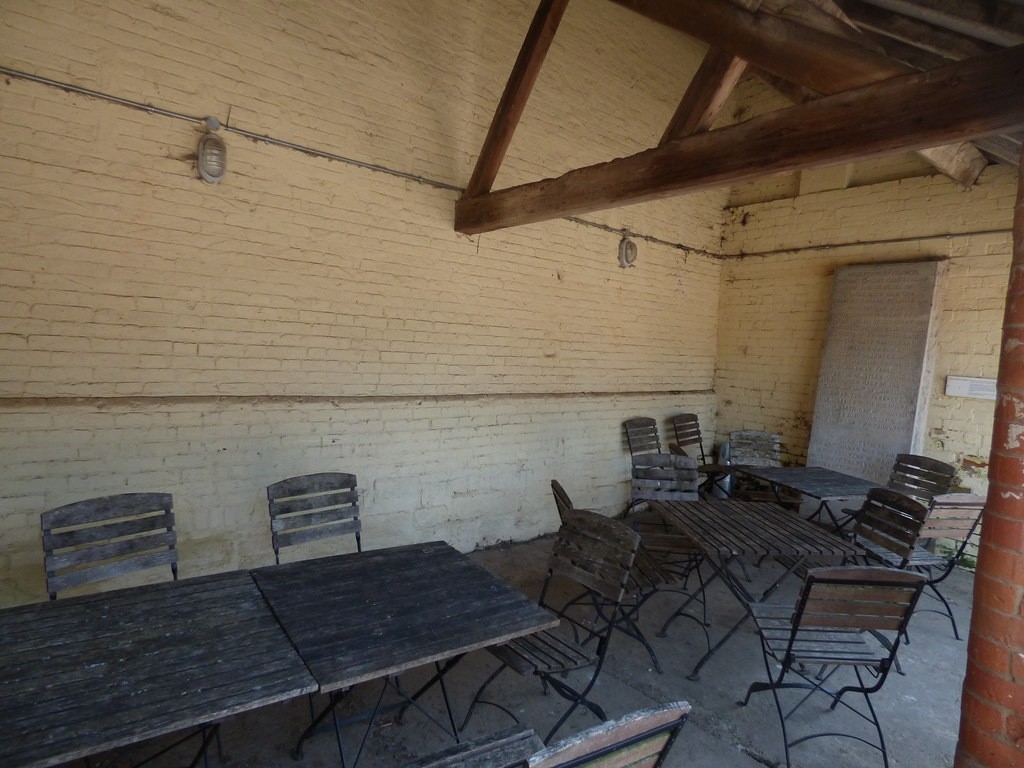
[459,508,642,747]
[377,701,691,767]
[267,472,405,724]
[549,412,986,768]
[40,492,236,766]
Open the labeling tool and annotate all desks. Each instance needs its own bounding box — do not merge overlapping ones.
[249,540,561,768]
[0,569,318,768]
[736,465,897,564]
[642,499,867,681]
